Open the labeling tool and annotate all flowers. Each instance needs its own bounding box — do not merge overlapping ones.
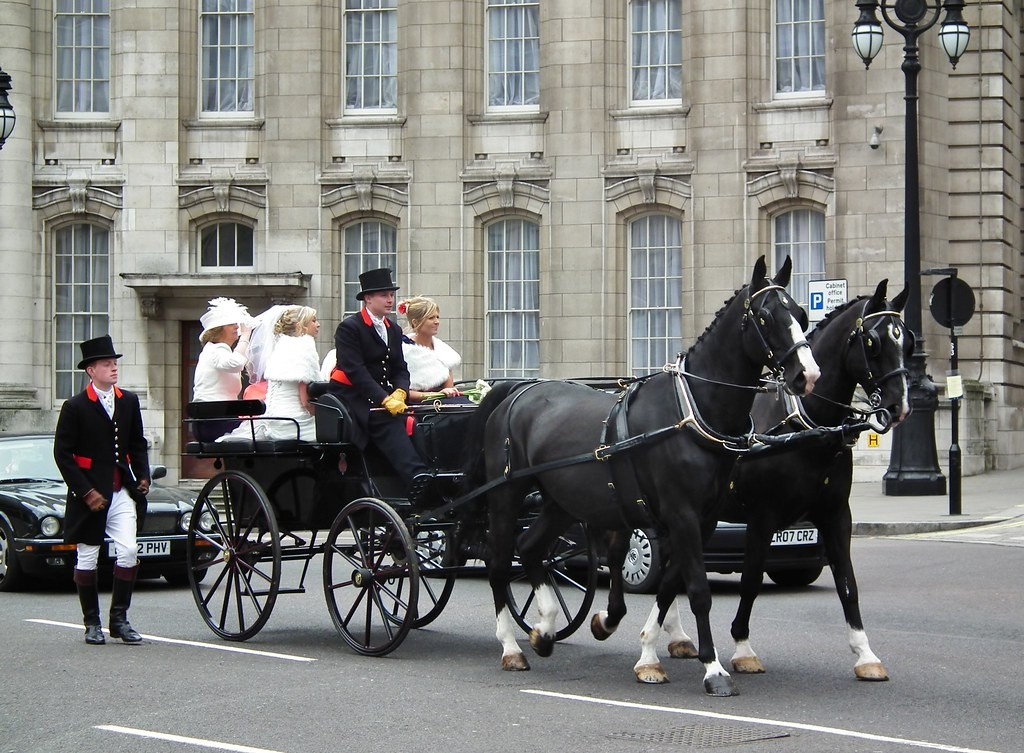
[419,379,492,405]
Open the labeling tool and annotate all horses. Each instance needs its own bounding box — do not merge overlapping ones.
[590,278,915,683]
[453,254,821,695]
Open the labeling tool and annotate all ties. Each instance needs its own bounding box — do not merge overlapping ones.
[377,321,386,343]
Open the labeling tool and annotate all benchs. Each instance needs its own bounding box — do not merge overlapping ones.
[307,380,478,443]
[183,395,316,458]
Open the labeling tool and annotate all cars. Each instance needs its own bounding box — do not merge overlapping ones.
[0,435,222,597]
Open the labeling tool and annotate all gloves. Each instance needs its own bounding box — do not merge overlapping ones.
[137,480,149,496]
[381,396,408,415]
[84,489,108,512]
[390,388,407,404]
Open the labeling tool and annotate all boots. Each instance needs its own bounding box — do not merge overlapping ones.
[74,565,105,644]
[109,558,142,641]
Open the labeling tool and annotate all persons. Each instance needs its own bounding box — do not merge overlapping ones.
[190,297,258,442]
[53,336,152,646]
[214,303,326,442]
[320,265,463,483]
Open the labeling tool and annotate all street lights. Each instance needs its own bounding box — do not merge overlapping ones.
[850,0,974,495]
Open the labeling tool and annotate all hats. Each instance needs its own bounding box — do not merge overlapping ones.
[356,268,400,300]
[78,337,123,369]
[198,297,261,343]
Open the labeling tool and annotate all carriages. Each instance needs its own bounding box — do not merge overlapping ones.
[180,254,917,700]
